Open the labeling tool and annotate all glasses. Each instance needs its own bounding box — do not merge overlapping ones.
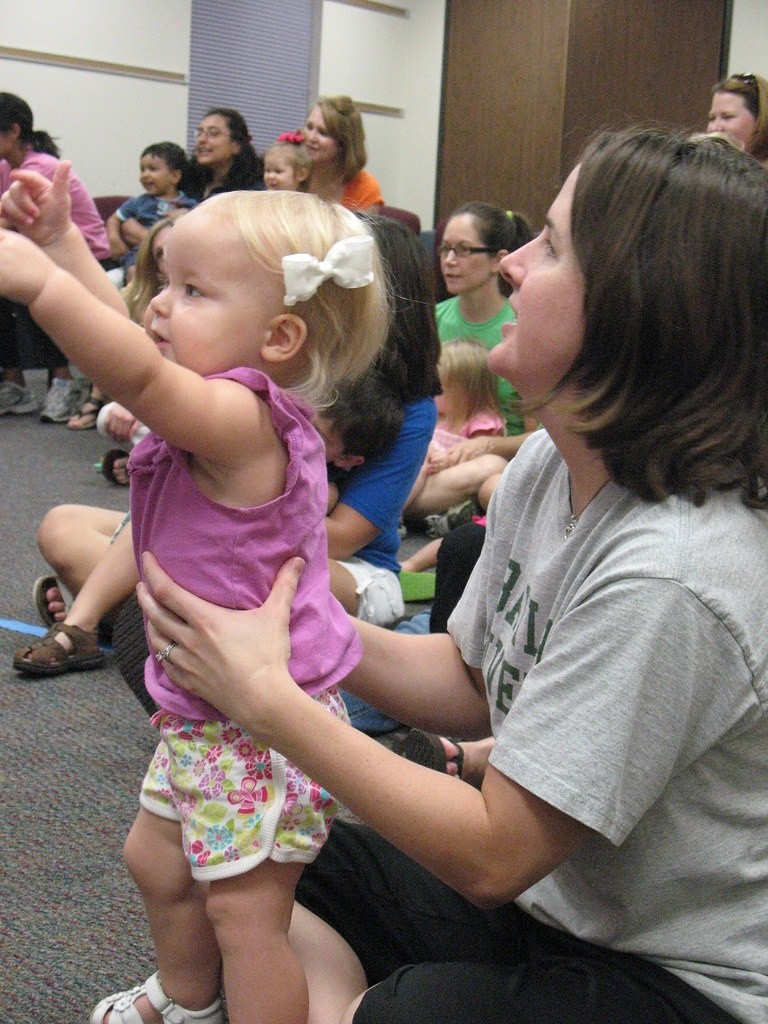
[436,243,499,257]
[731,73,758,91]
[194,128,232,139]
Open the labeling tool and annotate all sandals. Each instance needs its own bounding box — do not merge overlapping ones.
[401,727,464,782]
[110,590,163,734]
[13,622,103,674]
[90,971,224,1024]
[33,573,75,626]
[67,396,113,430]
[101,449,131,487]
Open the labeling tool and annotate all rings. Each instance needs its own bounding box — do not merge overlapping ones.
[155,644,176,661]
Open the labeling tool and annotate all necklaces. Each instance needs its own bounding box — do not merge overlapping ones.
[564,472,613,542]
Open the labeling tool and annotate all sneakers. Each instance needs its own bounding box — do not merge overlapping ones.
[0,381,37,416]
[40,378,90,424]
[425,500,482,540]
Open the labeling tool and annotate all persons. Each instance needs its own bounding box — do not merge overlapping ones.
[304,99,382,215]
[123,108,265,245]
[135,123,768,1024]
[0,160,391,1024]
[0,93,112,420]
[707,72,768,170]
[11,205,525,787]
